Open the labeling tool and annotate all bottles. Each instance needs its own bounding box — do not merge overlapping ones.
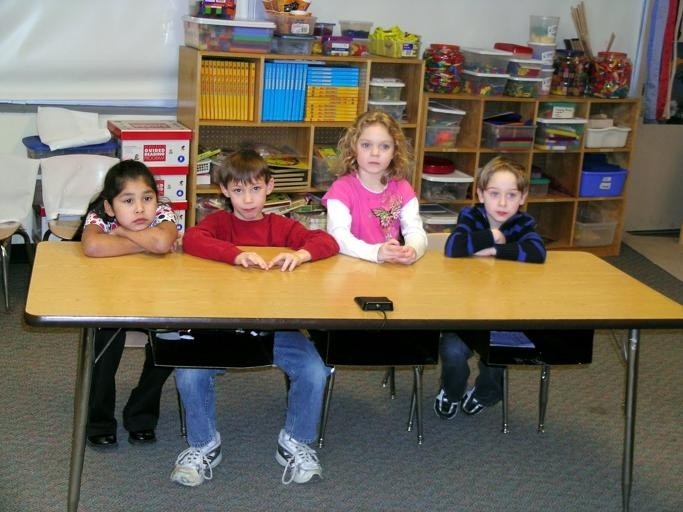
[422,44,464,92]
[590,51,631,99]
[550,50,591,95]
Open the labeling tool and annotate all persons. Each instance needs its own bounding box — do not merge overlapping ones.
[81,159,181,449]
[320,111,429,266]
[433,156,547,421]
[170,147,341,487]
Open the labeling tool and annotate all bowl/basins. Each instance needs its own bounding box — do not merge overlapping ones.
[527,14,560,95]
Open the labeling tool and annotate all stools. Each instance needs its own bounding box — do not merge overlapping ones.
[175,365,292,438]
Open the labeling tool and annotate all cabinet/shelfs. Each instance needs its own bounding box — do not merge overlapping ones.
[176,44,426,260]
[415,89,642,258]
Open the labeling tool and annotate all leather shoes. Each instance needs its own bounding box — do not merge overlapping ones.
[128,428,156,445]
[86,433,118,449]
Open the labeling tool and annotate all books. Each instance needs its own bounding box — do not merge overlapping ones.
[197,59,360,217]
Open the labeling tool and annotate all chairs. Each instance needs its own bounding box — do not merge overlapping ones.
[408,233,508,435]
[0,156,40,310]
[37,154,121,242]
[318,226,424,448]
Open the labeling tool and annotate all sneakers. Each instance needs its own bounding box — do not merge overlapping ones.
[276,429,323,484]
[432,386,488,420]
[170,432,222,486]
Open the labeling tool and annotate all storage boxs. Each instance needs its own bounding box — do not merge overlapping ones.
[274,32,317,57]
[105,119,193,167]
[460,43,546,98]
[23,134,120,205]
[312,152,338,191]
[169,202,190,237]
[30,203,83,241]
[339,19,373,39]
[421,101,631,247]
[182,15,276,55]
[145,167,189,202]
[367,34,424,61]
[368,78,406,102]
[288,209,326,232]
[367,98,407,123]
